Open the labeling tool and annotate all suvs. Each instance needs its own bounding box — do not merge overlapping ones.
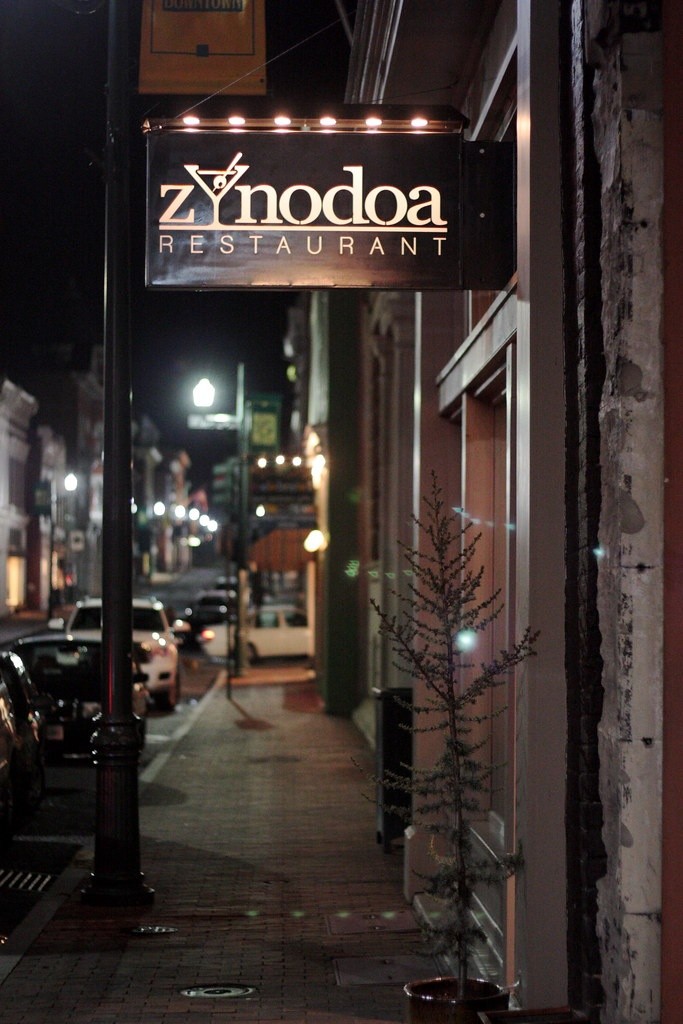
[48,598,190,712]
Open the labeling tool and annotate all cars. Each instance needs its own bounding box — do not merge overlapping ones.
[1,629,156,828]
[190,574,308,662]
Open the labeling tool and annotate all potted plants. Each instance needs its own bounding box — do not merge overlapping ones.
[348,470,542,1024]
